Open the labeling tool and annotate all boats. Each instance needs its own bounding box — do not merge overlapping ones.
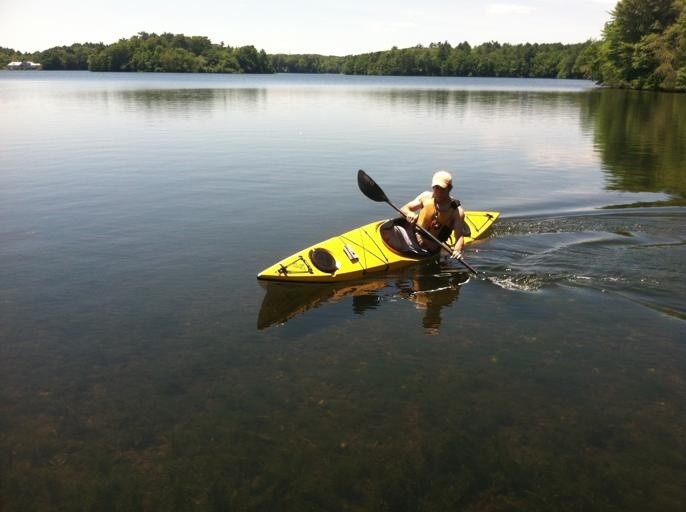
[256,211,501,283]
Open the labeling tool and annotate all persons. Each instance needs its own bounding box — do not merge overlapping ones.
[399,171,465,260]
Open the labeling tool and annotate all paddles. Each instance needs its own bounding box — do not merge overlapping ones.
[357,170,477,275]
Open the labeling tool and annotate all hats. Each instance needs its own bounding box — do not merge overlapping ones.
[431,171,452,189]
